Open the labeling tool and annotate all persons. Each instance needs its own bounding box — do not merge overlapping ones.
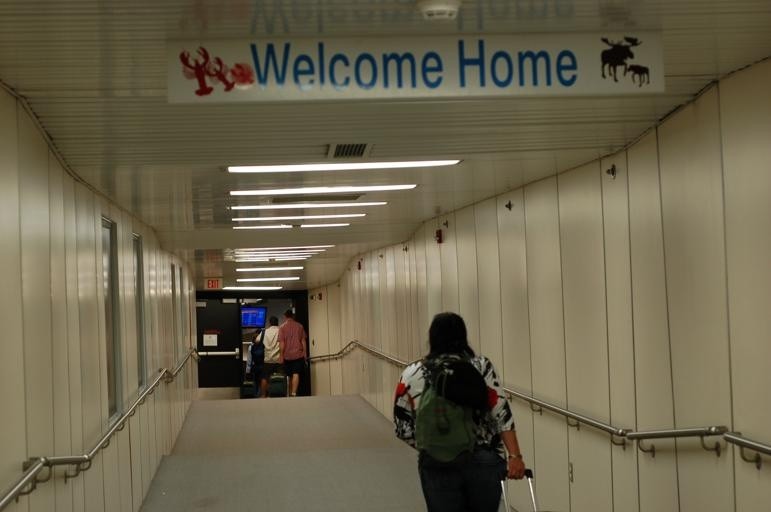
[245,309,306,398]
[394,312,524,512]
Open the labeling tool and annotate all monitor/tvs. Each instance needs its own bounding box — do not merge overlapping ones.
[242,305,268,329]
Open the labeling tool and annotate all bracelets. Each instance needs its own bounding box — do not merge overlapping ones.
[508,455,522,459]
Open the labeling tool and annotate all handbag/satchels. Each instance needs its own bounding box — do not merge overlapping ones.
[251,342,264,366]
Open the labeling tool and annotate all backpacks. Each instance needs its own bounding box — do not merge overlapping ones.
[416,355,476,463]
